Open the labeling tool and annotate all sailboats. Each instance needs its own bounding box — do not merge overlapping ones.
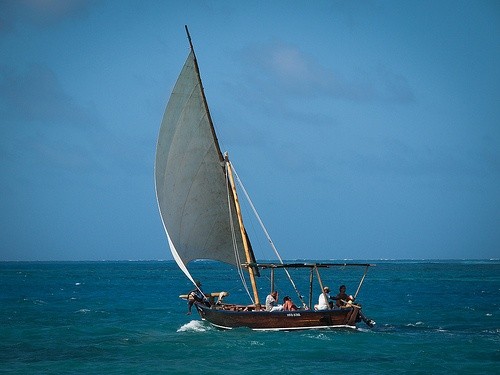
[154,24,376,331]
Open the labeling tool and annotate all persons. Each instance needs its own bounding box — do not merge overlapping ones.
[336,285,355,309]
[186,280,203,315]
[266,290,284,312]
[317,286,337,310]
[283,295,297,311]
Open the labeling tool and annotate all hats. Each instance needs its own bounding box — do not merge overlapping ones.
[323,287,329,290]
